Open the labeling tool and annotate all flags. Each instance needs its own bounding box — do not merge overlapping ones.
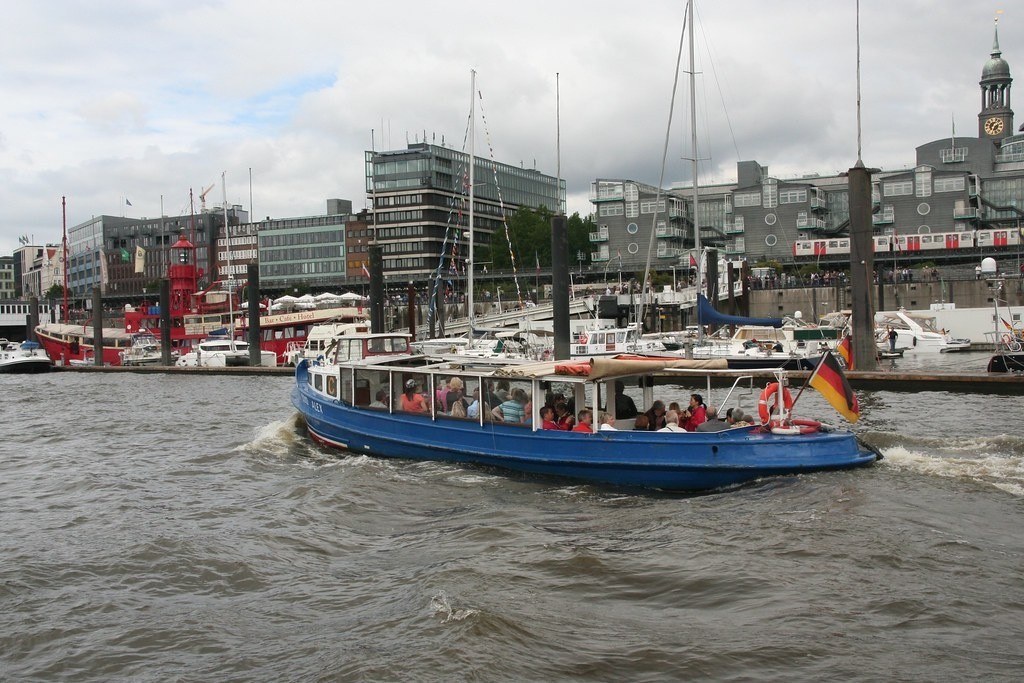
[126,199,132,206]
[362,265,371,280]
[86,246,91,251]
[690,255,699,269]
[808,352,860,425]
[1001,319,1012,332]
[26,237,31,243]
[838,333,855,372]
[23,236,27,241]
[20,238,25,245]
[120,246,130,262]
[1015,329,1024,340]
[135,246,146,273]
[426,91,544,338]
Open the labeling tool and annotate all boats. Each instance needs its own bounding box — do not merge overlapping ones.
[287,67,887,496]
[0,1,1024,386]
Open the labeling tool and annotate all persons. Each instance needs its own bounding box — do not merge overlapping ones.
[366,272,760,443]
[69,306,86,319]
[760,262,944,289]
[885,326,899,353]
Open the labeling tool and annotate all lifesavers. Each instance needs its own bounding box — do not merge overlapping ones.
[580,336,588,344]
[759,383,821,434]
[1002,333,1011,344]
